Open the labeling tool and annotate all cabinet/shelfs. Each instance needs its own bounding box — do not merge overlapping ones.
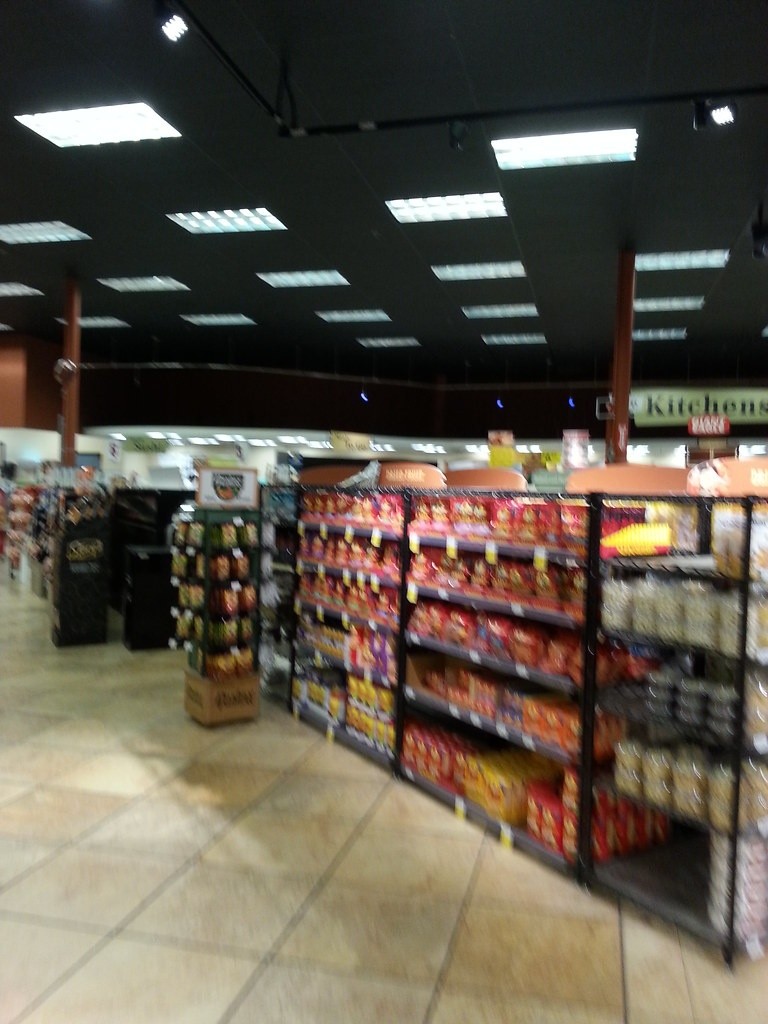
[261,482,768,971]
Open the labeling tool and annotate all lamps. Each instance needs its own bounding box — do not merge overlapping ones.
[693,96,739,131]
[751,203,768,259]
